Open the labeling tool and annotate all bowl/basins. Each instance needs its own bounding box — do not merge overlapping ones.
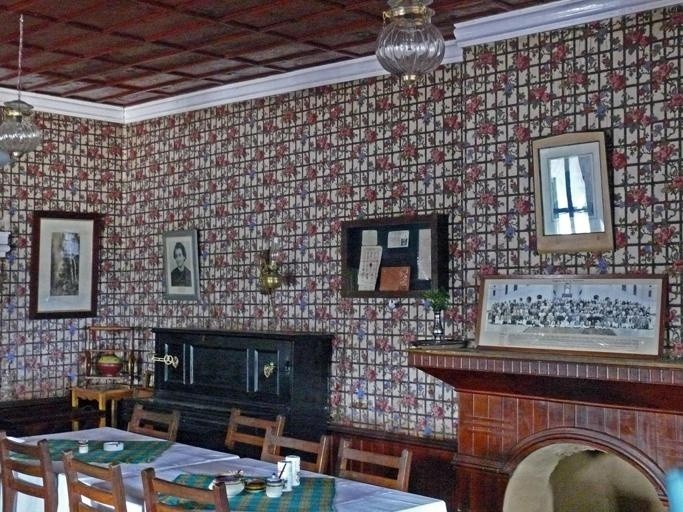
[97,361,123,377]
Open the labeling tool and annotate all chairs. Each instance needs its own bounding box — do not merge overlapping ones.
[126,404,181,441]
[137,467,231,512]
[260,425,334,476]
[0,429,58,512]
[338,439,413,492]
[226,406,285,462]
[62,448,131,511]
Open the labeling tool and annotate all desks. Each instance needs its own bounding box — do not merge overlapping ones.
[72,386,134,427]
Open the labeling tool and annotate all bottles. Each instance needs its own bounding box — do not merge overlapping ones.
[265,472,283,497]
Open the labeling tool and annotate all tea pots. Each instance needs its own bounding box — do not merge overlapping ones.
[206,478,245,499]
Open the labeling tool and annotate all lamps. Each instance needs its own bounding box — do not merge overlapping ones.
[0,11,42,160]
[373,0,445,85]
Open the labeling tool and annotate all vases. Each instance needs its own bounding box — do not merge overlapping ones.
[260,272,285,292]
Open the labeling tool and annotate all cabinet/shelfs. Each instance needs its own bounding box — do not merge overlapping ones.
[83,326,149,391]
[132,326,337,462]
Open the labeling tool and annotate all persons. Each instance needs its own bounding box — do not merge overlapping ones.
[171,241,190,286]
[486,292,654,329]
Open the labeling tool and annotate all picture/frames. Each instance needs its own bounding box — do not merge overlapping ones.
[161,230,201,303]
[28,208,104,320]
[337,211,451,300]
[476,275,667,362]
[530,129,616,259]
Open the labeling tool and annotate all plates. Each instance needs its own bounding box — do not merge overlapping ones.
[243,477,266,494]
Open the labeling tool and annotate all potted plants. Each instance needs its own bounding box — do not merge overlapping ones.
[424,287,453,346]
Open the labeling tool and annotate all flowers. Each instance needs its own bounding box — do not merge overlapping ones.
[254,243,291,273]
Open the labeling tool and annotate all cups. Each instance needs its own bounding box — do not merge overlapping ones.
[276,454,299,493]
[78,443,88,453]
